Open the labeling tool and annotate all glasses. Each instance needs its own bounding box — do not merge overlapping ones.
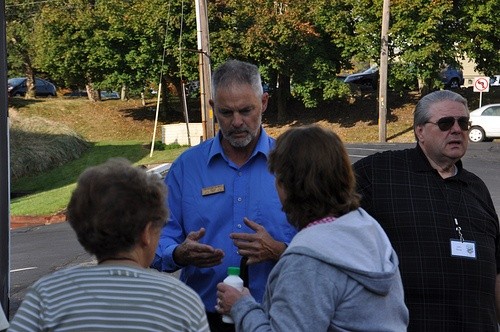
[425,116,473,131]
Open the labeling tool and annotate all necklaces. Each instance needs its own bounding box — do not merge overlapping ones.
[98,257,137,264]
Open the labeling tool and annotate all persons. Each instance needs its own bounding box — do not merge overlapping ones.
[214,125,410,332]
[6,157,211,332]
[352,90,500,332]
[150,60,299,332]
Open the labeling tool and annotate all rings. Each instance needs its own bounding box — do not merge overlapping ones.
[218,300,223,305]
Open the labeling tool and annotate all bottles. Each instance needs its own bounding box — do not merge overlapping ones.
[222,267,244,323]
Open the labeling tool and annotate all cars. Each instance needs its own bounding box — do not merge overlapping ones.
[100,91,120,100]
[334,61,466,98]
[467,103,500,143]
[8,77,57,98]
